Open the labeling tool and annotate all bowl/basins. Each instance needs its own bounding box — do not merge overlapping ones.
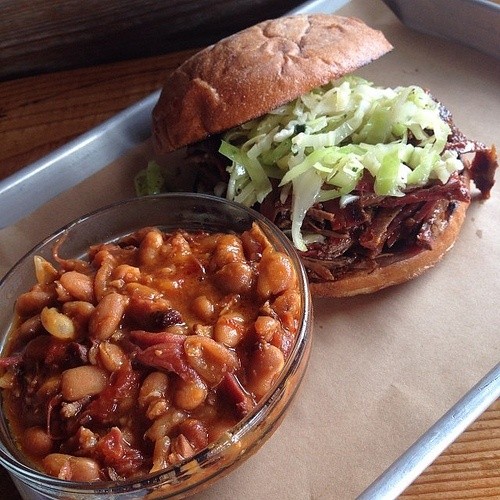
[0,193,314,500]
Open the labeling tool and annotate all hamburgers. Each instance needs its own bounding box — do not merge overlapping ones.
[134,15,500,298]
